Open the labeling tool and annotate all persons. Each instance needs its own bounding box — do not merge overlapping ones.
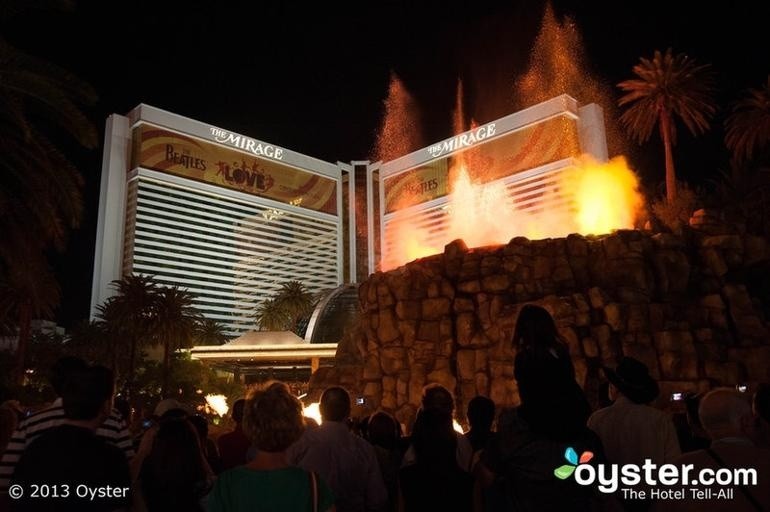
[506,304,593,437]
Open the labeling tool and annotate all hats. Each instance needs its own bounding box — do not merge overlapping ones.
[152,399,190,418]
[603,356,660,405]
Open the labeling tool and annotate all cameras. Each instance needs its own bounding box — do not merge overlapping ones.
[356,398,365,405]
[142,419,151,428]
[738,386,747,393]
[672,392,682,401]
